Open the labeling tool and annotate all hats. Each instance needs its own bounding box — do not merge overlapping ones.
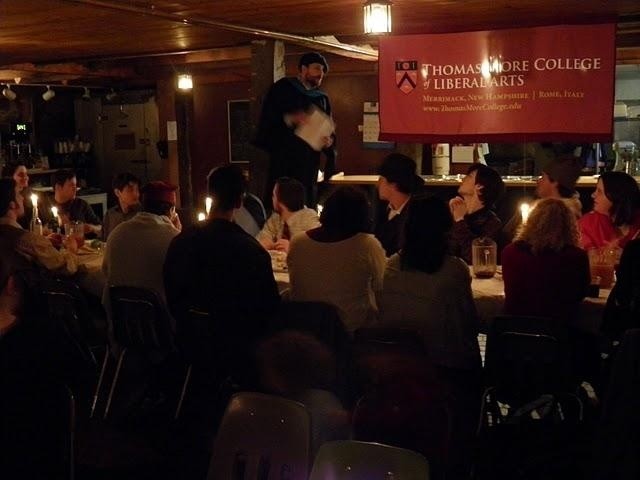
[375,152,425,188]
[296,51,328,73]
[141,178,177,203]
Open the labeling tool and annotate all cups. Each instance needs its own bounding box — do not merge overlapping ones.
[471,239,497,278]
[64,220,84,248]
[588,247,616,288]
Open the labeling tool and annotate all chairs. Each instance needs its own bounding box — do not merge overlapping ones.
[1,205,639,480]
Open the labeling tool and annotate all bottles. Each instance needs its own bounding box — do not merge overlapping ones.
[30,207,43,235]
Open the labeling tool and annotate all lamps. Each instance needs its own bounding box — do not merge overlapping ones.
[174,53,194,92]
[1,82,128,103]
[488,54,506,81]
[362,0,395,35]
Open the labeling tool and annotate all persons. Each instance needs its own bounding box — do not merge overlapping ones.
[248,52,336,207]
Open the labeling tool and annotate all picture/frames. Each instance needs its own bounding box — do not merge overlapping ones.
[226,97,261,164]
